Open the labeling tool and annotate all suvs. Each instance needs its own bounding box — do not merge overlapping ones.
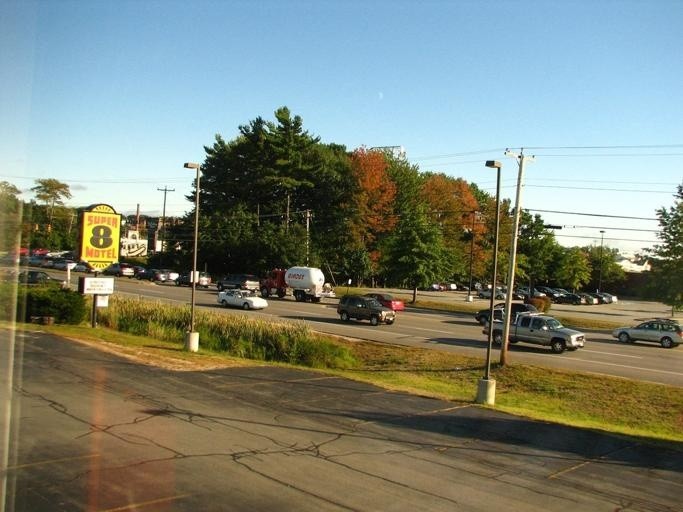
[609,318,682,349]
[211,271,260,292]
[332,293,395,327]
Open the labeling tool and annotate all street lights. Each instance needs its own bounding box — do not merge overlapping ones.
[473,158,503,407]
[182,161,204,353]
[598,229,605,293]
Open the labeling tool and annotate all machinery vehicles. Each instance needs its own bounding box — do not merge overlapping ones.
[258,264,325,304]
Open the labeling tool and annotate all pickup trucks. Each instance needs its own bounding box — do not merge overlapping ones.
[482,311,586,354]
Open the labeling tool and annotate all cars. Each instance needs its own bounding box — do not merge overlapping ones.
[471,301,539,325]
[362,291,404,312]
[213,288,270,311]
[475,284,620,305]
[0,240,214,293]
